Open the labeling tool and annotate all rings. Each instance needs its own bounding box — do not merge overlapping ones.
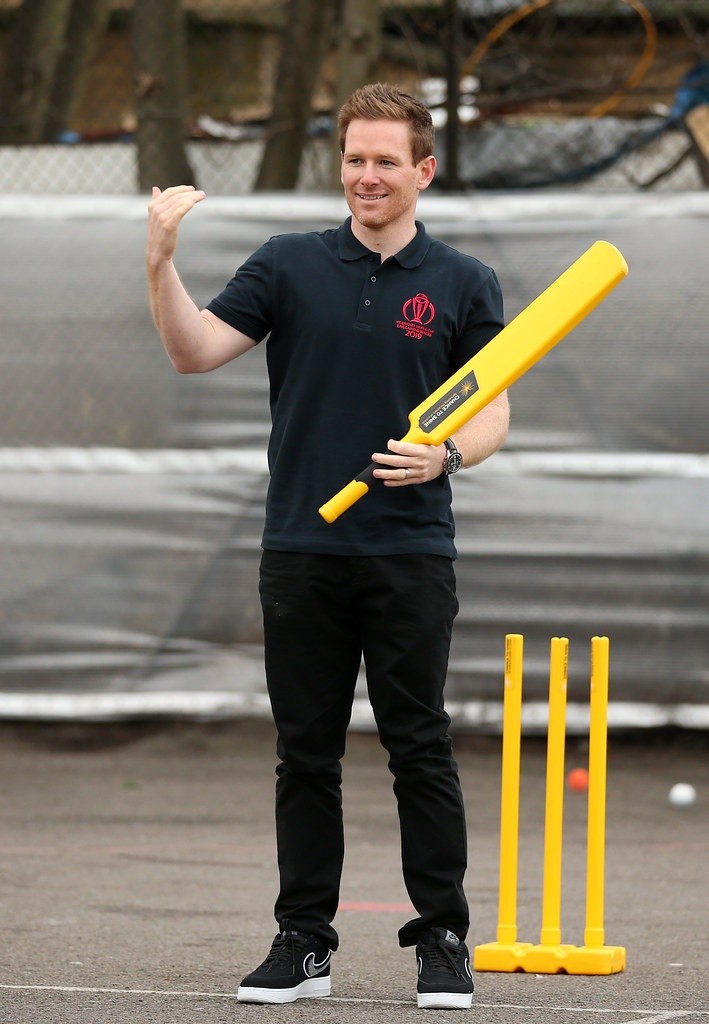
[406,468,410,479]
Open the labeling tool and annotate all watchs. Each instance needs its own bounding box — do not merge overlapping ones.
[444,439,463,474]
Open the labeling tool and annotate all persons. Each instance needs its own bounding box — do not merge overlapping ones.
[149,83,510,1009]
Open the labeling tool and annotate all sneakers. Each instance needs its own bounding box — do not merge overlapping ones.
[416,938,474,1009]
[237,918,331,1003]
